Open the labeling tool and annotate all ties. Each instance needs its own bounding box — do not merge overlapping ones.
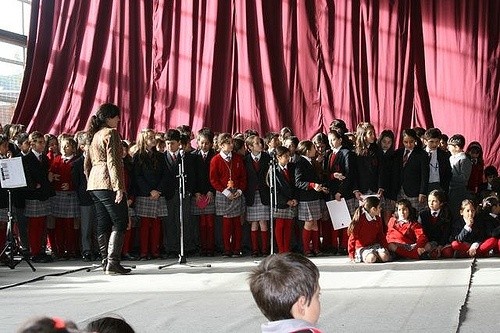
[329,153,335,167]
[432,211,437,221]
[226,157,231,162]
[254,158,259,172]
[282,168,289,182]
[172,152,177,161]
[403,150,410,166]
[203,154,207,161]
[38,154,43,164]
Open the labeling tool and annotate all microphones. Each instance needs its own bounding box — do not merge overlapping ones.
[179,148,186,158]
[270,148,277,156]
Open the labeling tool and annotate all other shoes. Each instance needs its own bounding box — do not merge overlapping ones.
[454,251,465,258]
[305,249,344,256]
[232,254,241,258]
[222,253,231,257]
[262,250,269,257]
[58,254,67,261]
[251,249,260,257]
[33,254,53,263]
[121,253,135,261]
[430,247,441,259]
[79,249,96,262]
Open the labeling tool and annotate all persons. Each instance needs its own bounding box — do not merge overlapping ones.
[247,252,325,333]
[82,317,136,333]
[84,103,132,274]
[119,119,500,264]
[0,115,100,262]
[17,314,81,333]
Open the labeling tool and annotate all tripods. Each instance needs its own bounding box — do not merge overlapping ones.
[0,157,37,271]
[158,156,211,269]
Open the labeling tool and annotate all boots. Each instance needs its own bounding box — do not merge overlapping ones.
[105,232,131,275]
[98,234,110,275]
